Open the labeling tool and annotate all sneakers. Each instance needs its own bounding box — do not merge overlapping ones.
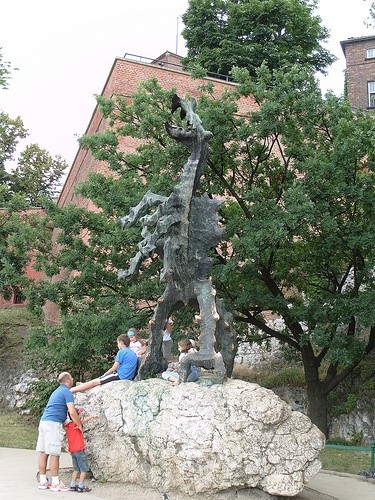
[49,480,70,492]
[37,479,52,490]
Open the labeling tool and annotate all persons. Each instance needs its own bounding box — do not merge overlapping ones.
[127,327,146,357]
[162,318,174,341]
[70,334,137,393]
[64,408,99,492]
[36,372,83,492]
[161,339,200,386]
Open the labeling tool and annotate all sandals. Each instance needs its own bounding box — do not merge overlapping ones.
[70,486,78,491]
[77,486,91,492]
